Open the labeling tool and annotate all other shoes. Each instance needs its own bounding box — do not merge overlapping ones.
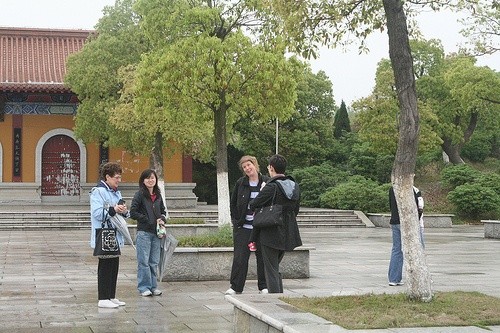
[139,290,153,297]
[260,289,269,293]
[111,298,126,306]
[388,280,404,286]
[225,288,235,294]
[97,299,119,308]
[153,289,162,296]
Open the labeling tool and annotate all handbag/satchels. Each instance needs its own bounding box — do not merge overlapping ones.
[252,185,283,227]
[92,208,121,257]
[156,221,166,239]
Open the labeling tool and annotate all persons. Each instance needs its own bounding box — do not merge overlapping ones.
[89,162,130,308]
[131,169,167,298]
[226,155,272,296]
[386,185,425,287]
[249,154,302,293]
